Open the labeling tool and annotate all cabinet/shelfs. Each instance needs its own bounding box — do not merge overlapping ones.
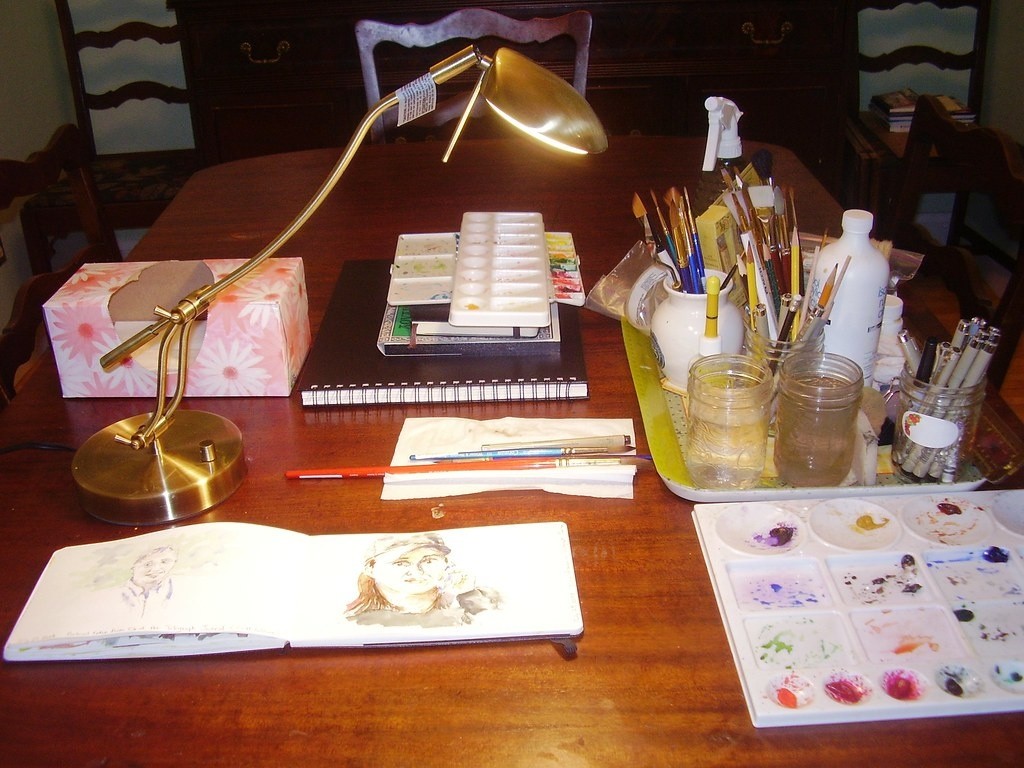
[167,0,849,204]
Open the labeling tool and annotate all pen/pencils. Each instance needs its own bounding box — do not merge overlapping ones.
[897,316,1001,485]
[819,263,839,306]
[747,240,758,333]
[791,227,800,341]
[762,238,781,315]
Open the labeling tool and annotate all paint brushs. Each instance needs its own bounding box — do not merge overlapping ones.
[632,188,743,289]
[284,434,650,478]
[717,161,851,438]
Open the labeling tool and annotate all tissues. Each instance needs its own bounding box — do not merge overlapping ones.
[41,256,313,399]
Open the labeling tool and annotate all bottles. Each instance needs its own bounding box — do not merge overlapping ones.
[807,210,889,398]
[875,295,903,383]
[652,268,746,394]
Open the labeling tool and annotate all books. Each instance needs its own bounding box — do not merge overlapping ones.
[3,520,584,662]
[869,87,977,134]
[299,251,593,408]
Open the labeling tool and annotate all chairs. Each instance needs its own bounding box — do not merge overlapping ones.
[354,8,592,145]
[845,0,1024,393]
[0,0,198,414]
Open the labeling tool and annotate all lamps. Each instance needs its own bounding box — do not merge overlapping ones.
[71,45,612,526]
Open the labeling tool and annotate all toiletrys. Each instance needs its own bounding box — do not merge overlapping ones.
[691,94,750,220]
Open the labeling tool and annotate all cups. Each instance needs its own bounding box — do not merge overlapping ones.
[774,350,863,485]
[683,354,775,489]
[889,360,987,484]
[742,316,825,440]
